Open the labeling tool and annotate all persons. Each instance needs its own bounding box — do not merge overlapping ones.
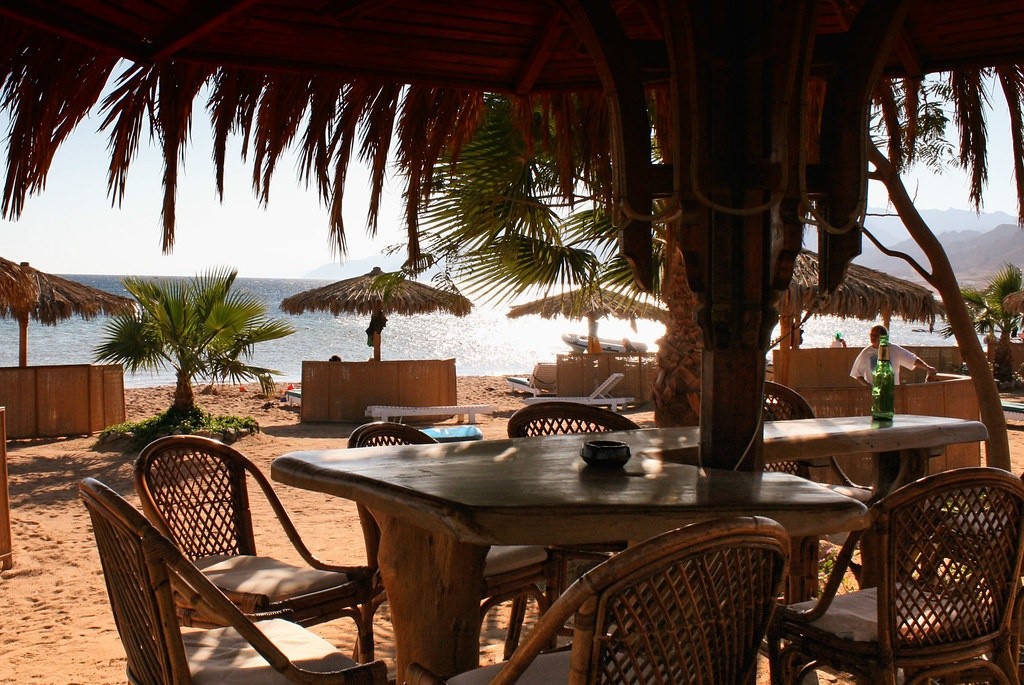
[848,325,937,387]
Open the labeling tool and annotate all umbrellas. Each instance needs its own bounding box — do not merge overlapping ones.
[506,284,673,353]
[0,2,1024,507]
[280,267,476,362]
[0,258,138,331]
[772,246,946,350]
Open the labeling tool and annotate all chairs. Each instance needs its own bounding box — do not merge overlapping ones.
[78,477,388,685]
[348,421,552,663]
[503,402,641,661]
[767,468,1024,685]
[506,362,557,397]
[758,380,874,660]
[447,516,792,685]
[133,435,374,667]
[524,373,635,413]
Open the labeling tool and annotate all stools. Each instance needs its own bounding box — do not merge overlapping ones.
[1001,401,1024,420]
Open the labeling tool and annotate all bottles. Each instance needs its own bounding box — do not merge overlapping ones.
[871,336,895,429]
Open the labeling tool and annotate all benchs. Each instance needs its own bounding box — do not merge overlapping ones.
[365,405,492,423]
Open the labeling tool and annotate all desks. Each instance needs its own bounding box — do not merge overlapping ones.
[270,414,990,685]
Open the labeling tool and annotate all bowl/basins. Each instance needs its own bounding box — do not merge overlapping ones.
[579,439,631,468]
